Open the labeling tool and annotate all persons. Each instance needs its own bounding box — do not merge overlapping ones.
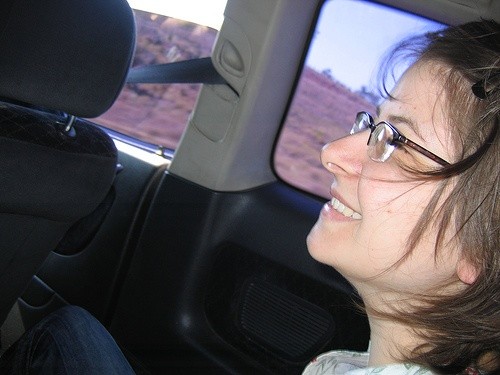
[0,17,500,375]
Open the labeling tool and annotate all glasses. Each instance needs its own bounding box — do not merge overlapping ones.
[349,111,452,168]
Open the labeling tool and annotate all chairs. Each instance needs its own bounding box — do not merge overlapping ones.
[0,0,137,327]
